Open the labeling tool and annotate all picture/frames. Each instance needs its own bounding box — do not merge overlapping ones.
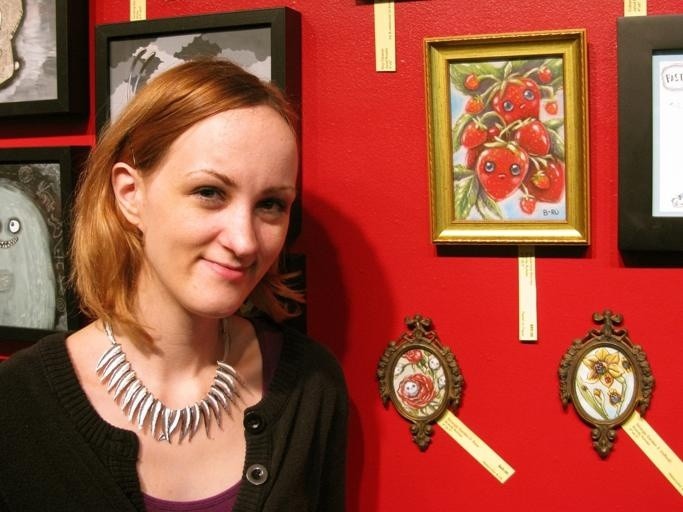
[615,14,682,258]
[91,5,306,142]
[2,139,93,341]
[421,28,597,252]
[0,0,91,138]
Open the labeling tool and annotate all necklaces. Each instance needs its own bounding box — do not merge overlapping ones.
[93,311,246,445]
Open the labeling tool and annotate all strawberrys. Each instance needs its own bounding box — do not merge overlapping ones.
[461,66,564,215]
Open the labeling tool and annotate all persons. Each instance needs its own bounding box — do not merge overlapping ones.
[1,59,348,511]
